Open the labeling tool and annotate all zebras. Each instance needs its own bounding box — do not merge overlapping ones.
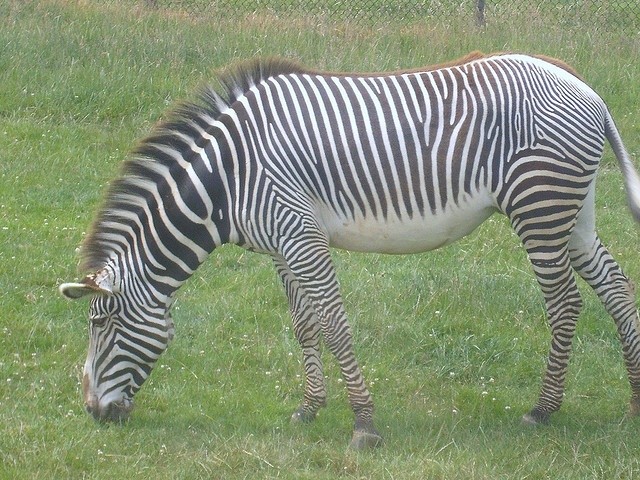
[59,56,640,455]
